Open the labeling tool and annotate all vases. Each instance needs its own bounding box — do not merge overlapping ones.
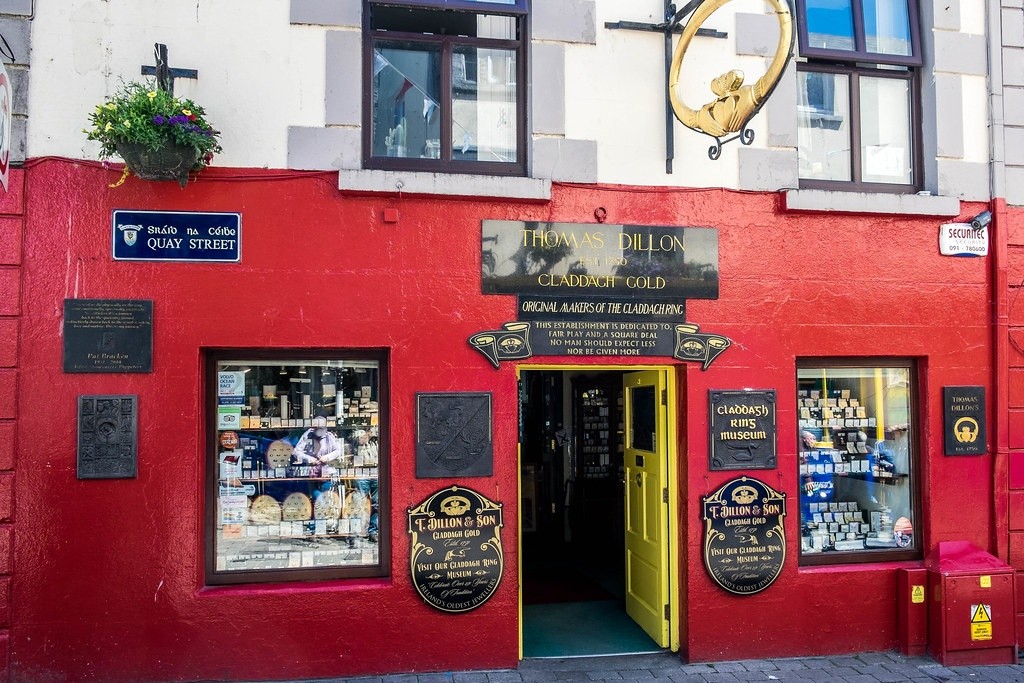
[111,137,195,173]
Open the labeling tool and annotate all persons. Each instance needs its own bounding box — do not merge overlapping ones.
[269,416,379,549]
[865,437,896,509]
[799,427,833,537]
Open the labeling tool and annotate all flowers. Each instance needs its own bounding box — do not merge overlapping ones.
[80,76,222,190]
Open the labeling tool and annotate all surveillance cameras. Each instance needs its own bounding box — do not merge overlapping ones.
[972,210,992,230]
